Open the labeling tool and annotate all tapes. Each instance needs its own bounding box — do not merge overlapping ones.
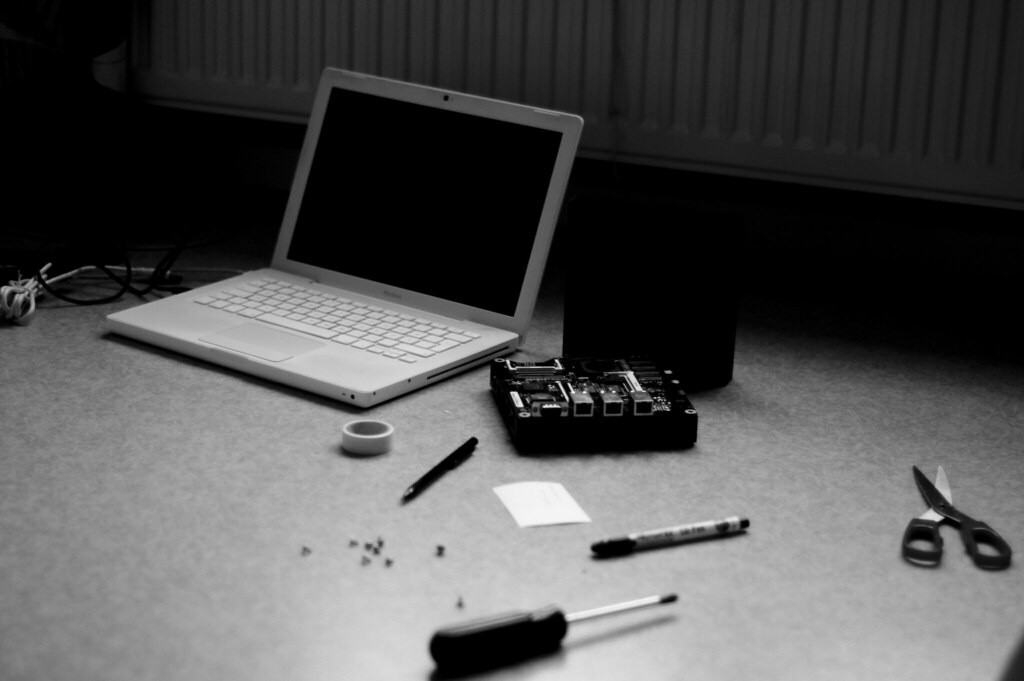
[342,417,400,456]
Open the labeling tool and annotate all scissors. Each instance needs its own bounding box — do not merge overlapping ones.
[900,460,1016,573]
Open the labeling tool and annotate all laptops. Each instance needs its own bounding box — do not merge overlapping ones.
[106,67,585,408]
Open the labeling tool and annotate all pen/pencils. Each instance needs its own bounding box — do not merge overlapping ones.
[590,514,752,561]
[396,434,478,507]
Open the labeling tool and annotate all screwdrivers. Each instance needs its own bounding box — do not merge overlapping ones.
[430,588,678,672]
